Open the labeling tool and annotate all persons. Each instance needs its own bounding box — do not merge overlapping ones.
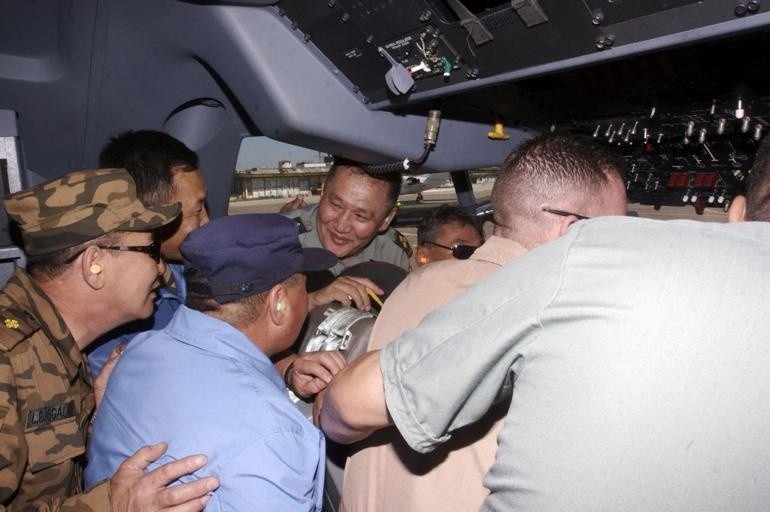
[313,134,770,512]
[80,128,306,377]
[0,168,219,511]
[273,161,415,311]
[85,211,346,512]
[341,136,627,512]
[410,207,480,268]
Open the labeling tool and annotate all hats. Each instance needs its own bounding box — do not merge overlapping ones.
[177,213,340,305]
[2,166,183,257]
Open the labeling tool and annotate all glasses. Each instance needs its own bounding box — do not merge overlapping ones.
[419,240,480,260]
[61,237,162,265]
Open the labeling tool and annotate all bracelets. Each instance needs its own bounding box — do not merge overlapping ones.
[281,360,294,386]
[318,406,322,428]
